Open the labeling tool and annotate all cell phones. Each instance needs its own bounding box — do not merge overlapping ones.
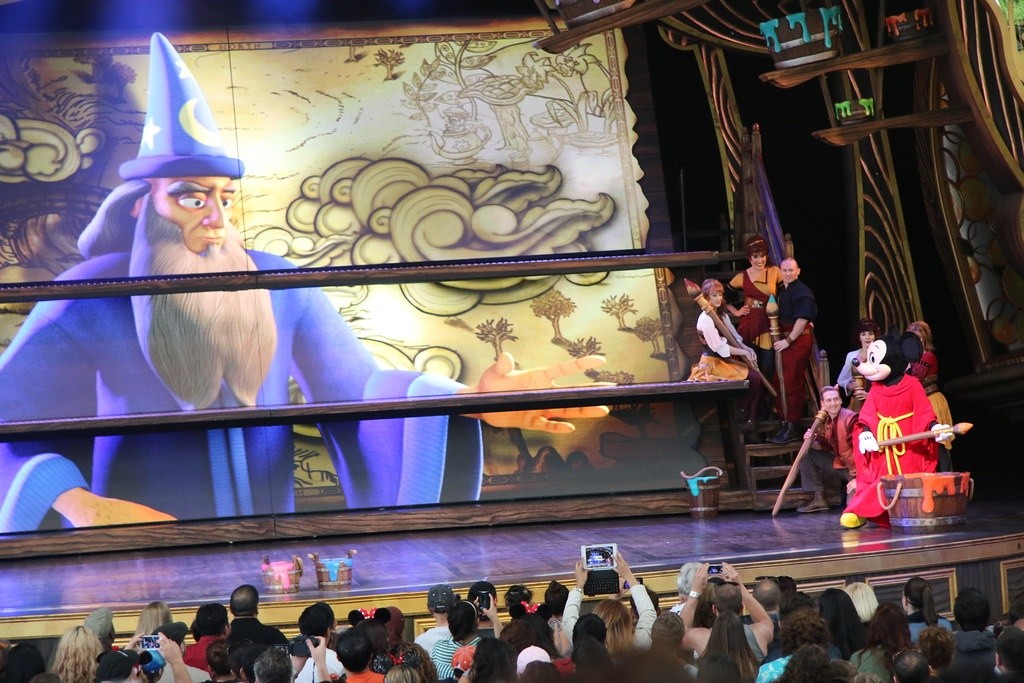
[478,591,491,620]
[140,635,162,650]
[707,564,725,575]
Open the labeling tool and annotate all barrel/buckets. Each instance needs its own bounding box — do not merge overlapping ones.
[876,471,975,536]
[308,550,356,591]
[686,471,720,520]
[261,555,303,593]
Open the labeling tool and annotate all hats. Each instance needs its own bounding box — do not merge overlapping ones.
[650,611,685,646]
[427,583,456,613]
[452,645,476,671]
[516,645,552,675]
[150,622,188,647]
[84,608,114,639]
[94,649,140,681]
[141,649,167,673]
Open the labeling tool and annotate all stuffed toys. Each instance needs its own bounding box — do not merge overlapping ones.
[840,331,955,529]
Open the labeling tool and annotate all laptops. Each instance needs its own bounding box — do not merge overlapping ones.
[581,543,619,597]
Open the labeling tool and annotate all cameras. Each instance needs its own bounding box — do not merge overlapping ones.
[273,634,318,657]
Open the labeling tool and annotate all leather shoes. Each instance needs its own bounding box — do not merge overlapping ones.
[796,490,830,513]
[765,420,803,444]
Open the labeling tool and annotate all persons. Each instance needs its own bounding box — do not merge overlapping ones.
[796,318,879,513]
[696,235,817,443]
[906,321,952,472]
[0,552,1024,683]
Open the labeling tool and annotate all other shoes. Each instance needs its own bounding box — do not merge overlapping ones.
[734,409,761,443]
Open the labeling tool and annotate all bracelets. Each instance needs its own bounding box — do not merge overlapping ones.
[574,588,583,593]
[689,591,701,599]
[785,336,793,344]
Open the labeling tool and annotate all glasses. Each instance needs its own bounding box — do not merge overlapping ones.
[716,581,741,594]
[753,576,779,586]
[226,639,255,658]
[892,646,923,668]
